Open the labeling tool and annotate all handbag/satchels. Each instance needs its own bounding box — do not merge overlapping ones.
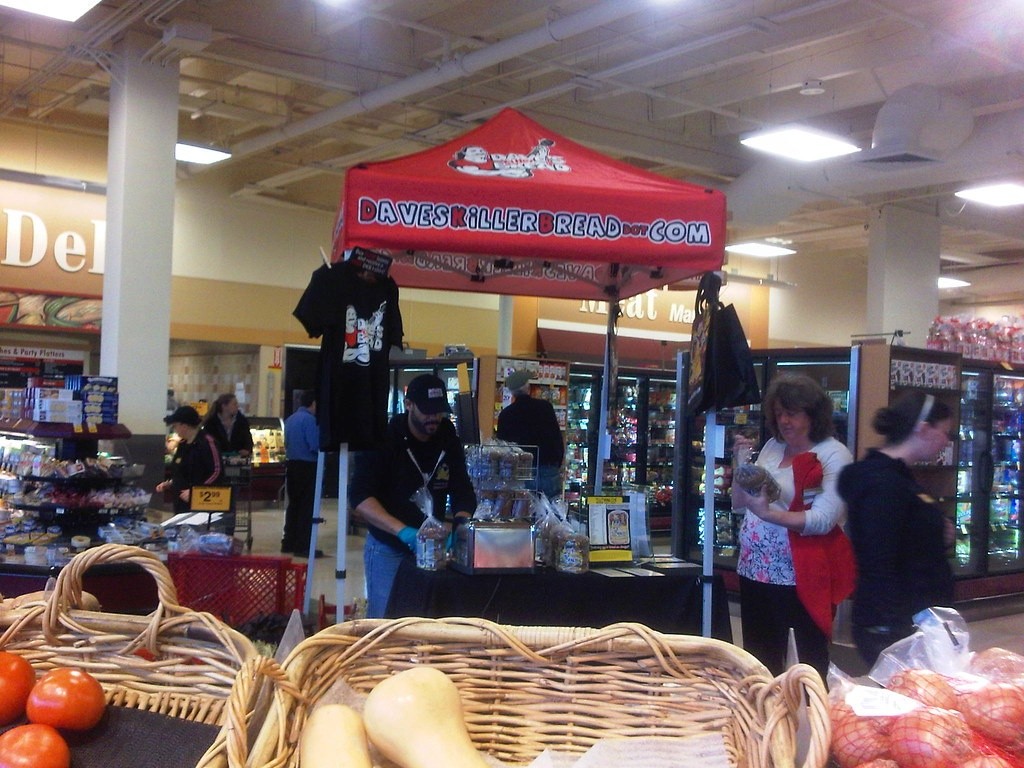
[687,274,762,416]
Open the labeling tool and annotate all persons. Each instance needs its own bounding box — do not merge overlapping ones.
[497,371,565,523]
[156,394,253,537]
[837,389,954,674]
[281,391,324,559]
[731,373,854,706]
[349,374,478,618]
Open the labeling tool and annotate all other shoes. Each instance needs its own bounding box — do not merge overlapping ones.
[281,545,323,558]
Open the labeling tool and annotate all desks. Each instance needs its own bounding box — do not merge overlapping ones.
[385,553,734,645]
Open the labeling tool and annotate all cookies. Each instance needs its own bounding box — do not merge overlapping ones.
[85,384,116,423]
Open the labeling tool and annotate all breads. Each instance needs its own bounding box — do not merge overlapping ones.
[736,465,779,500]
[418,526,449,570]
[469,448,590,576]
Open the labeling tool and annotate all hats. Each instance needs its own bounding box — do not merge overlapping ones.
[407,374,453,415]
[164,406,198,424]
[507,370,532,390]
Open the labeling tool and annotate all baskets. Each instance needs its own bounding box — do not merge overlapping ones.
[225,616,832,768]
[0,543,266,768]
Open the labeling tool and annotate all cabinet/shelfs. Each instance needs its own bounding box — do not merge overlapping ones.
[478,354,571,502]
[445,444,540,523]
[0,417,243,615]
[545,340,1024,624]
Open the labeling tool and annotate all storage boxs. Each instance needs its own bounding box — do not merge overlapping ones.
[9,376,118,426]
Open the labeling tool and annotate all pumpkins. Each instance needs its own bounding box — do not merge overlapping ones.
[295,667,492,768]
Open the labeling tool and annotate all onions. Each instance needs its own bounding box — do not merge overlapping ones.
[833,647,1024,768]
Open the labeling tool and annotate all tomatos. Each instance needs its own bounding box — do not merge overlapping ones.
[0,650,106,768]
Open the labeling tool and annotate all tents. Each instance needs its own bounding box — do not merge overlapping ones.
[303,106,728,637]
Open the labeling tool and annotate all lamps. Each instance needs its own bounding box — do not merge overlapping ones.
[738,122,863,163]
[176,139,232,165]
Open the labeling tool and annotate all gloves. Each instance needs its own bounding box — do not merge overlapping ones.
[397,526,418,554]
